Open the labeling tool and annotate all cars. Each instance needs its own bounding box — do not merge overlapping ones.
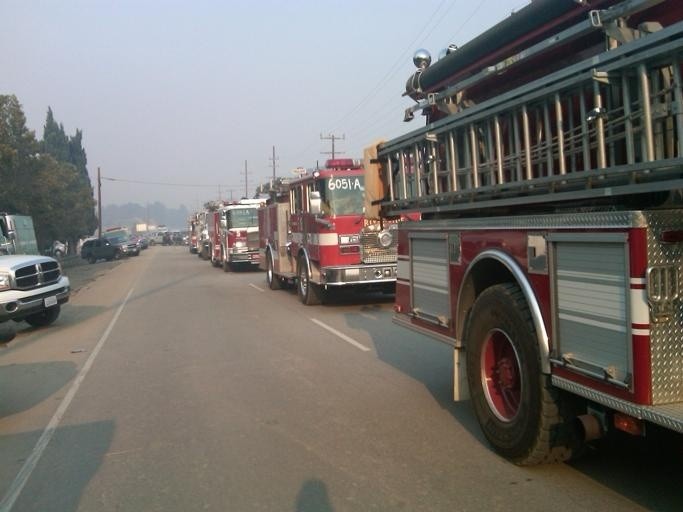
[80,229,179,264]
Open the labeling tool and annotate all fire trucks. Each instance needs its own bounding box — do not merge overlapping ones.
[267,159,397,304]
[378,0,683,467]
[187,198,267,271]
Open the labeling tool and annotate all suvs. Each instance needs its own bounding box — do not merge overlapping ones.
[0,213,71,329]
